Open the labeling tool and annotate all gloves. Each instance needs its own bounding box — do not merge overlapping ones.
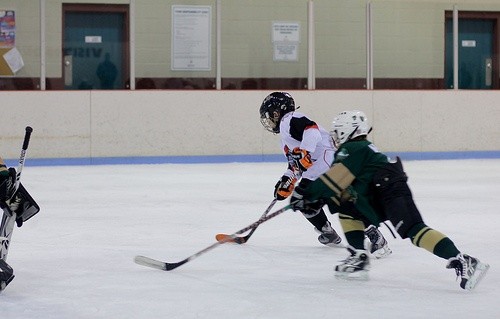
[274,169,298,200]
[290,178,325,215]
[291,147,314,173]
[0,168,22,218]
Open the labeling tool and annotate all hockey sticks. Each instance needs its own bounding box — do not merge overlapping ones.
[134,200,301,271]
[215,198,277,245]
[0,126,33,237]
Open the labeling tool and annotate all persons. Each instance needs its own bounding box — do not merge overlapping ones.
[96,53,116,89]
[0,156,40,291]
[260,92,393,259]
[302,110,490,291]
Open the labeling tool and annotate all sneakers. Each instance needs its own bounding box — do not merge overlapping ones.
[334,246,370,281]
[445,253,490,291]
[314,222,344,248]
[363,225,393,259]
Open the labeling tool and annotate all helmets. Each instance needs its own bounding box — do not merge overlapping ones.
[259,92,300,134]
[328,110,373,151]
